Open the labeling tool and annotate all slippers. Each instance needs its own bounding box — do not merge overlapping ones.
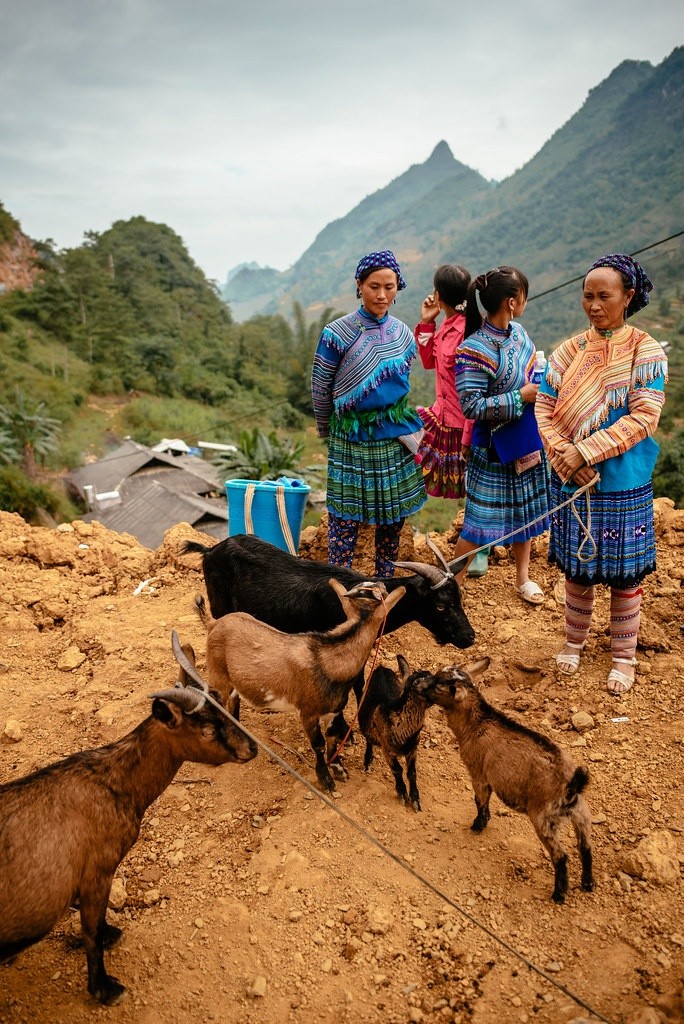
[514,582,546,603]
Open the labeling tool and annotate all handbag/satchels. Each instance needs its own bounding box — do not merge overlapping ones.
[397,427,434,465]
[514,450,542,476]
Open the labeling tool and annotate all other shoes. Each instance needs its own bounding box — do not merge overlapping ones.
[466,546,491,577]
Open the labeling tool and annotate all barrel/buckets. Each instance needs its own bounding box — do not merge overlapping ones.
[224,479,312,557]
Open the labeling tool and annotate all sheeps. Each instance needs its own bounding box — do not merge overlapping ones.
[2,632,260,1009]
[410,654,594,905]
[182,530,476,810]
[446,690,449,692]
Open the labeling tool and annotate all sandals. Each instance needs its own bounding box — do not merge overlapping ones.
[607,658,640,696]
[556,640,586,676]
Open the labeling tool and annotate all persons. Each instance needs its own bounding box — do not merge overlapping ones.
[311,251,433,577]
[450,266,551,604]
[534,254,667,696]
[413,265,491,577]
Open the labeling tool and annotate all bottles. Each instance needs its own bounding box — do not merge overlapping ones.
[533,351,548,384]
